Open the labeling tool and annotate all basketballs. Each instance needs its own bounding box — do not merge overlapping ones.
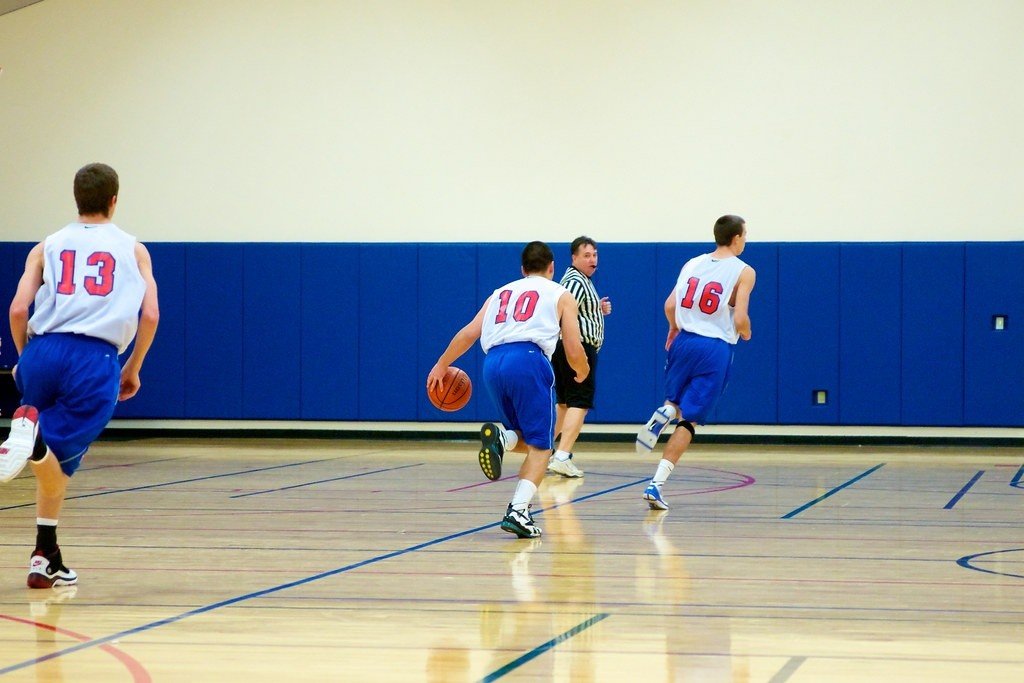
[426,366,473,413]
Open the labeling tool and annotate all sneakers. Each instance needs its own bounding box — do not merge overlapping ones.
[26,544,77,588]
[547,453,584,478]
[501,503,543,538]
[643,481,669,510]
[636,408,672,454]
[0,406,40,483]
[478,423,505,481]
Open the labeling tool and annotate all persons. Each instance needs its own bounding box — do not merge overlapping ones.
[0,163,160,590]
[551,237,611,479]
[427,241,590,539]
[635,216,756,511]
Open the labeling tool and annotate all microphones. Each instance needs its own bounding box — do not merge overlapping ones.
[593,265,597,268]
